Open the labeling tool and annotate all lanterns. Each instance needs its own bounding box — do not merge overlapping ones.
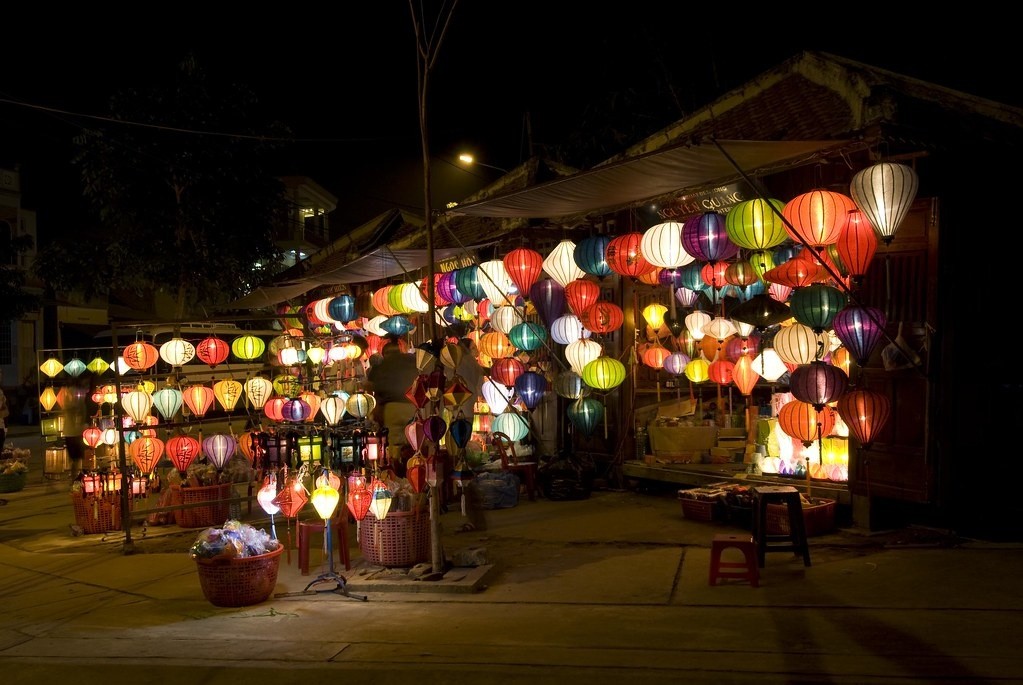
[231,160,920,565]
[39,337,264,498]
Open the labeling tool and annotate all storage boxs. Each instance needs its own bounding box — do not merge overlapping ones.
[648,420,748,465]
[678,497,836,538]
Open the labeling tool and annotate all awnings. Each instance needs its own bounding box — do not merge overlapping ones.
[271,242,567,371]
[202,282,322,336]
[434,139,917,367]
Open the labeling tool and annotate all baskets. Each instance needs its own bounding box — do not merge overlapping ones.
[677,496,718,521]
[765,496,837,538]
[723,494,763,527]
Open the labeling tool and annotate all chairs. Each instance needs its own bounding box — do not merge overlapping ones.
[493,432,537,502]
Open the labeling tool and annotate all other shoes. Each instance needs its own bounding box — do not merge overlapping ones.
[0,498,9,506]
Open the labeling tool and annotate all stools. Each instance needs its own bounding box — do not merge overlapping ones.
[709,534,759,587]
[298,519,350,576]
[752,486,811,566]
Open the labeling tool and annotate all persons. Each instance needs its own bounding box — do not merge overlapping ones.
[330,334,369,424]
[0,389,9,451]
[85,383,113,468]
[62,386,88,483]
[367,343,420,457]
